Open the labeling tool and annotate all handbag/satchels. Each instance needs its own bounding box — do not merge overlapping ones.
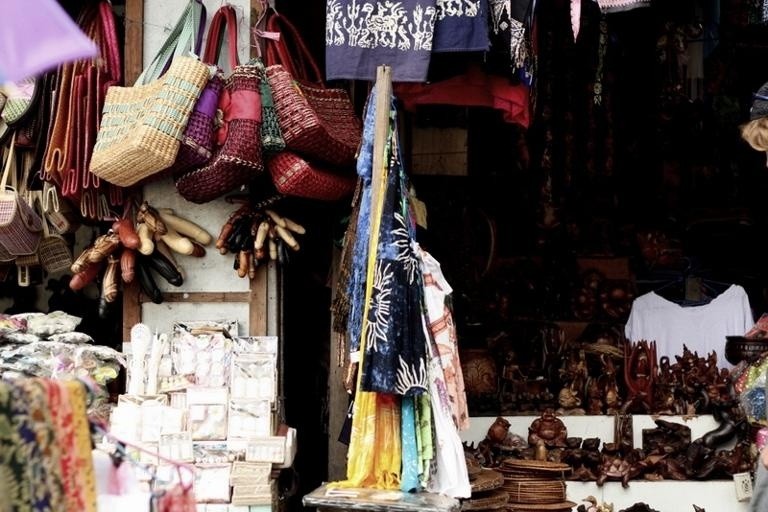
[1,0,127,277]
[90,1,367,199]
